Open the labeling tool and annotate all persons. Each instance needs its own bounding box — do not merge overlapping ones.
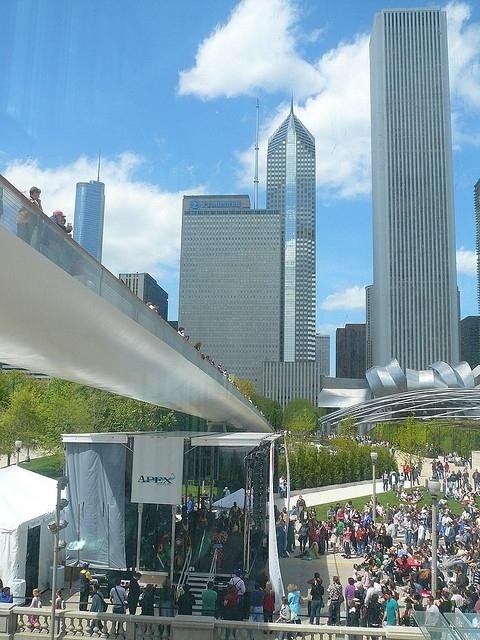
[1,459,480,639]
[147,302,160,314]
[42,210,73,264]
[177,327,253,405]
[15,187,42,245]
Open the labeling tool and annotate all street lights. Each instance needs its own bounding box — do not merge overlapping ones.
[369,448,378,528]
[280,429,293,533]
[15,440,22,465]
[426,473,443,600]
[47,473,70,640]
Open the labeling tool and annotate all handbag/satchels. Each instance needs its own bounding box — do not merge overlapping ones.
[122,603,126,613]
[320,600,324,609]
[102,601,108,612]
[338,595,344,603]
[299,596,308,606]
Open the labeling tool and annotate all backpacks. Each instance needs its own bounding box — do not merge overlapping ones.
[60,599,66,610]
[327,599,333,607]
[228,579,241,592]
[464,575,470,586]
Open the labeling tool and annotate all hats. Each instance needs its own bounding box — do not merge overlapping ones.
[233,568,244,574]
[81,562,89,568]
